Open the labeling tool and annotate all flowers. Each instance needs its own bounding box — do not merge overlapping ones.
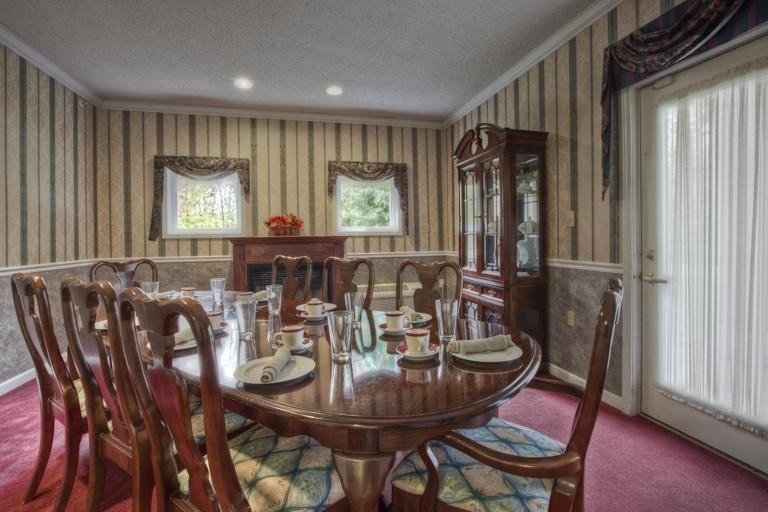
[265,212,304,227]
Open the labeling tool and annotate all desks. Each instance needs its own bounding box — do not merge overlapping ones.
[94,288,543,512]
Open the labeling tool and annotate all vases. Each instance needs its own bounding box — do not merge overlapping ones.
[270,226,299,236]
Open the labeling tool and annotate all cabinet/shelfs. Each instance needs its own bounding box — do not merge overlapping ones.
[450,123,549,375]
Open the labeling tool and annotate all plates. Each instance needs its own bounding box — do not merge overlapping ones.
[147,339,200,350]
[272,339,314,352]
[396,342,440,361]
[233,355,316,385]
[379,335,406,342]
[296,312,327,321]
[449,341,523,363]
[402,312,432,322]
[379,323,413,337]
[256,292,275,302]
[213,329,227,335]
[94,315,143,331]
[296,303,337,311]
[451,363,526,376]
[397,358,441,369]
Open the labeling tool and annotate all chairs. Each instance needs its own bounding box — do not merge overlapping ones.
[13,271,110,511]
[56,278,156,512]
[89,258,160,303]
[393,260,464,332]
[271,256,313,297]
[118,283,252,511]
[319,258,375,310]
[396,277,623,512]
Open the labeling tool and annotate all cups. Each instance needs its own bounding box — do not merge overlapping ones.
[210,278,226,305]
[344,292,364,322]
[385,312,402,329]
[329,363,354,404]
[406,370,431,388]
[305,325,324,338]
[351,324,366,361]
[237,342,257,365]
[235,302,256,340]
[141,280,159,298]
[236,292,253,303]
[406,329,430,354]
[208,310,222,328]
[266,285,283,313]
[275,326,305,346]
[306,301,327,315]
[267,315,282,342]
[387,341,401,354]
[181,287,196,298]
[212,306,224,323]
[438,340,461,382]
[327,311,353,361]
[435,299,458,338]
